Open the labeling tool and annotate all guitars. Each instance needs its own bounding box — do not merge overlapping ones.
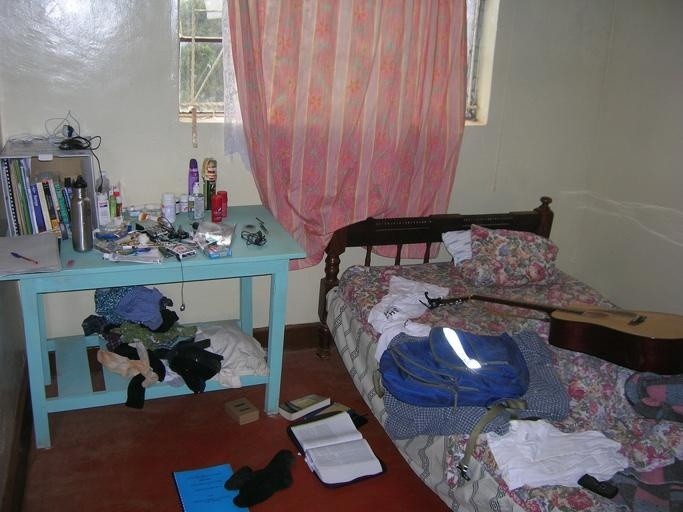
[419,292,682,375]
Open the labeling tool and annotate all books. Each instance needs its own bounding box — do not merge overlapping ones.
[283,410,383,486]
[172,463,250,511]
[278,392,330,421]
[0,158,74,237]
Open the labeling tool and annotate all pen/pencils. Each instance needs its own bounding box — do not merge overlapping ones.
[10,252,38,265]
[256,216,268,234]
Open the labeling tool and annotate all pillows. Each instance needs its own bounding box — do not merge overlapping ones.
[442,223,558,288]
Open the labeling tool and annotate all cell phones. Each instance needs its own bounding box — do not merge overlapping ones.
[577,474,620,499]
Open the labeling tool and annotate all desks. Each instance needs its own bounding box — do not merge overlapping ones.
[1,204,307,449]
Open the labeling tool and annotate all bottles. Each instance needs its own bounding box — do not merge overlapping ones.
[161,192,180,223]
[71,179,92,252]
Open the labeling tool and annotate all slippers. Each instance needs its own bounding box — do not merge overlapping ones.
[224,448,296,507]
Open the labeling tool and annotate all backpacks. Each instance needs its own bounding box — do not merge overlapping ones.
[380,326,529,406]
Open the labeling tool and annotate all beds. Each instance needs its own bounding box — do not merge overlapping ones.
[317,197,683,512]
